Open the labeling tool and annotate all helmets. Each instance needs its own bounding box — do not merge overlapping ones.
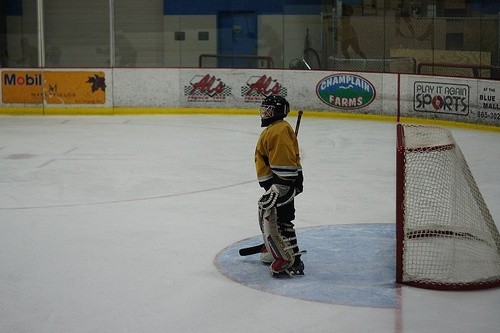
[259,95,290,127]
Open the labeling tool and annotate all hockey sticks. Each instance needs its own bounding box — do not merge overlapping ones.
[239,110,303,255]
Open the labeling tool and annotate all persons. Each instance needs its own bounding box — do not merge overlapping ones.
[17,4,500,82]
[254,93,305,276]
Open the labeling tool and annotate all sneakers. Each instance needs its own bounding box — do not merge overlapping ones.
[272,259,305,277]
